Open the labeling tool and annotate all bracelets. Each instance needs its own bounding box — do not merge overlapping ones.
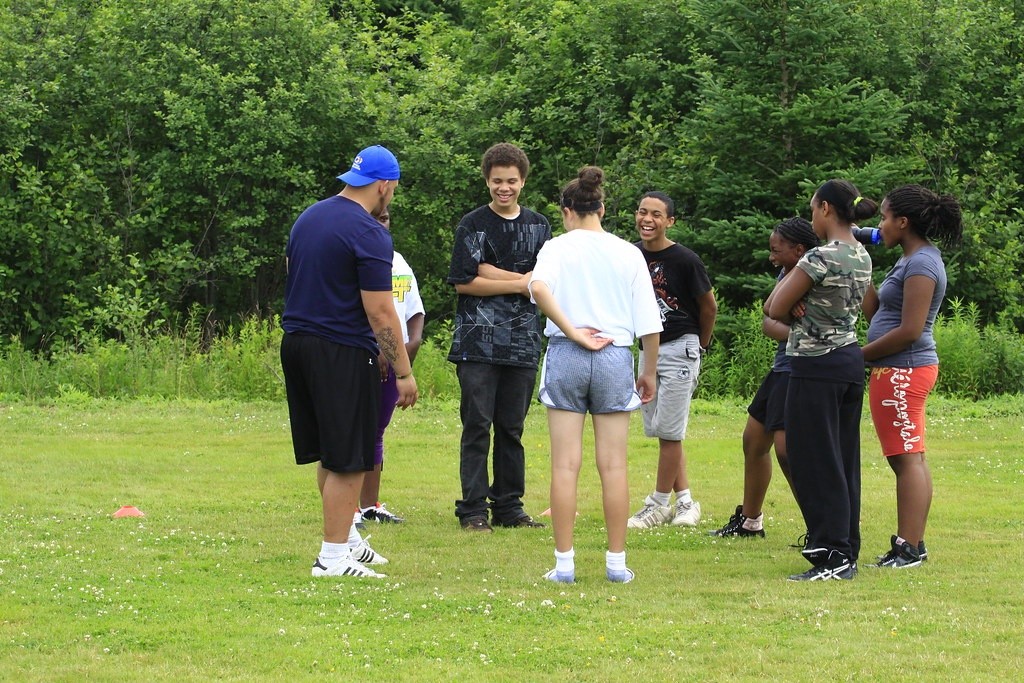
[396,369,412,379]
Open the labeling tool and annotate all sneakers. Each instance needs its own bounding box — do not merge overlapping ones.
[628,495,673,529]
[862,534,921,569]
[350,509,366,531]
[505,513,544,528]
[671,495,702,527]
[543,569,574,585]
[875,540,928,560]
[606,567,635,585]
[704,504,766,538]
[852,563,858,580]
[312,550,388,579]
[786,561,854,582]
[786,528,810,550]
[358,503,402,524]
[461,516,494,534]
[350,535,389,564]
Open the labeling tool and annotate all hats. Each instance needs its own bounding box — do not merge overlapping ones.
[335,144,401,186]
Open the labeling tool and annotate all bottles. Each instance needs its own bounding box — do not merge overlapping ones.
[852,227,880,244]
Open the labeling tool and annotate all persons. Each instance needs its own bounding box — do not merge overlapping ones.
[445,143,553,532]
[528,165,664,586]
[280,145,418,576]
[763,178,872,581]
[626,191,717,528]
[351,203,426,526]
[703,216,820,550]
[860,184,963,568]
